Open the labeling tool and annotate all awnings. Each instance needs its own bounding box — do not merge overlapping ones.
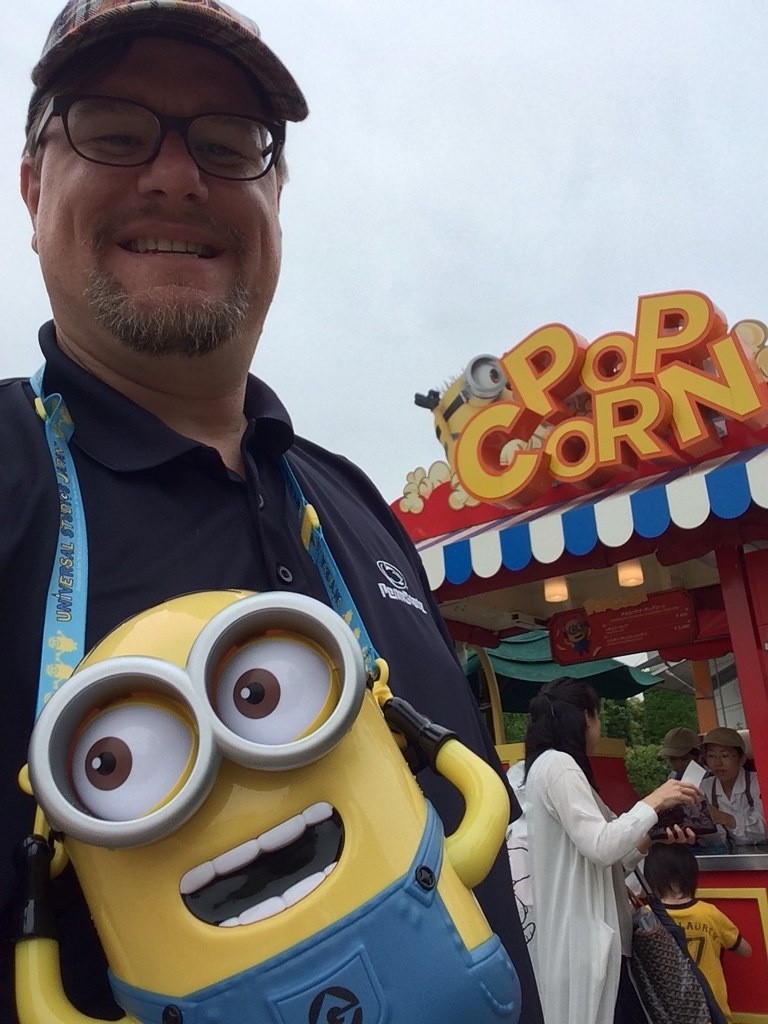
[465,630,665,714]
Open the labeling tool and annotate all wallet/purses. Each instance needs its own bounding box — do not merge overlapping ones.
[647,792,717,839]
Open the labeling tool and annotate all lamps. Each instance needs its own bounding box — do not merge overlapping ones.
[617,559,644,587]
[544,576,568,603]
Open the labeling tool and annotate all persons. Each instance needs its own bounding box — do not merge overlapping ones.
[0,0,549,1024]
[505,678,767,1024]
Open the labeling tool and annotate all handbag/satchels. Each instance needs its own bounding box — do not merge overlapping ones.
[612,883,713,1024]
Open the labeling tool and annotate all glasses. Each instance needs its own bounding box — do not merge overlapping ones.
[30,88,288,182]
[704,749,740,760]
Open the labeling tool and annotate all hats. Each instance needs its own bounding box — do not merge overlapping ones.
[694,725,746,760]
[25,0,308,123]
[658,727,703,757]
